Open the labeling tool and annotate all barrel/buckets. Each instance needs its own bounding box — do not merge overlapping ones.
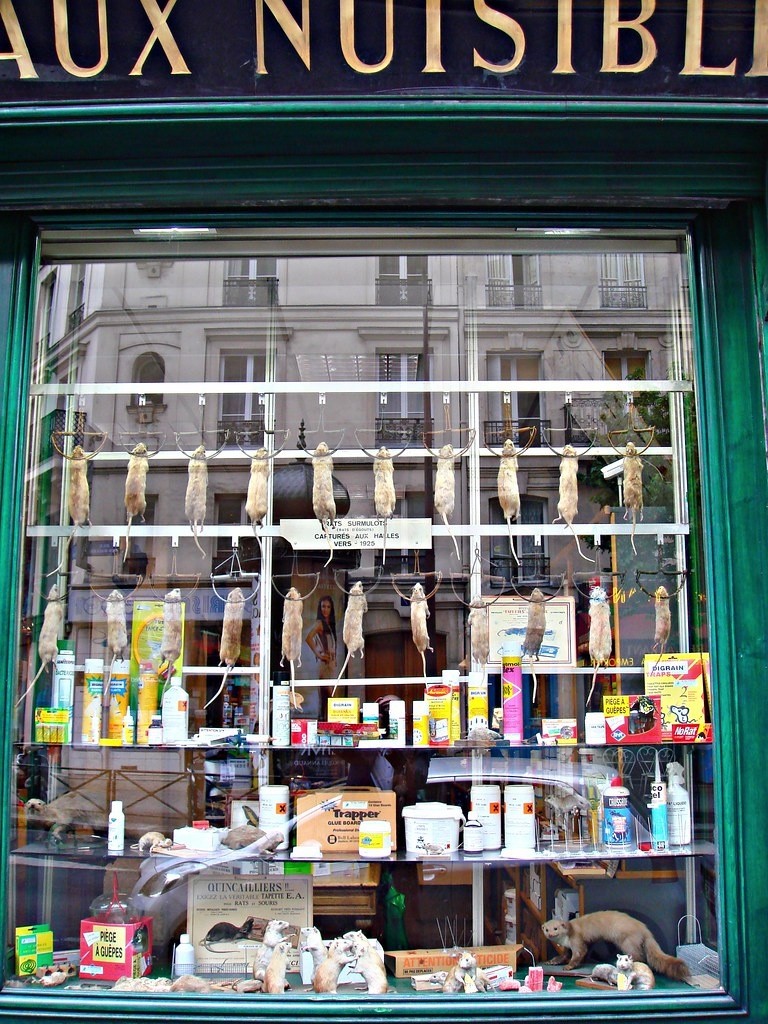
[504,888,516,918]
[505,914,516,943]
[504,938,516,945]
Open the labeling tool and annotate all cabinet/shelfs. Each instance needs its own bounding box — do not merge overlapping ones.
[9,381,725,999]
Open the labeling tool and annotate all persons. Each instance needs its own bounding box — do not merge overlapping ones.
[304,596,336,722]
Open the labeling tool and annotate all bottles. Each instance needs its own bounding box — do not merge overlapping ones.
[176,933,195,976]
[148,715,163,746]
[602,776,632,848]
[137,659,158,746]
[667,776,691,845]
[389,700,406,744]
[463,811,483,854]
[413,700,429,746]
[50,639,73,742]
[108,800,125,850]
[162,677,189,743]
[363,702,379,729]
[584,712,606,744]
[82,658,104,744]
[107,659,130,738]
[272,671,291,746]
[122,706,134,744]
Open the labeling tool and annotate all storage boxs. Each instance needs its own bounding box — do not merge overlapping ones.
[79,918,152,981]
[295,789,397,852]
